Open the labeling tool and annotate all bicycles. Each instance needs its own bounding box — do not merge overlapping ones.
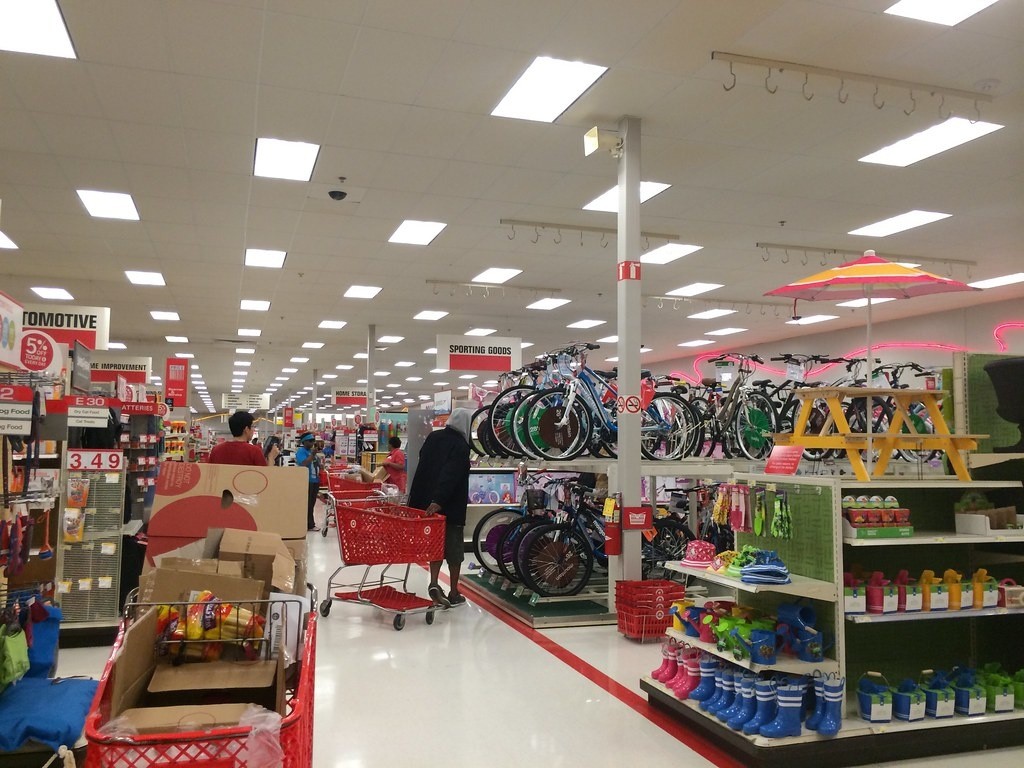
[467,341,943,464]
[471,485,500,504]
[471,468,734,597]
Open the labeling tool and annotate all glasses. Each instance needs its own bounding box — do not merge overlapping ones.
[305,439,315,443]
[251,425,257,428]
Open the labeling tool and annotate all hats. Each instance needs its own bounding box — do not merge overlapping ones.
[724,544,760,577]
[680,540,716,568]
[706,550,738,574]
[314,436,324,442]
[740,549,792,584]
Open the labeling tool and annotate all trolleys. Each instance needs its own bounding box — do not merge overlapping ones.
[84,586,318,768]
[321,469,382,537]
[320,489,451,631]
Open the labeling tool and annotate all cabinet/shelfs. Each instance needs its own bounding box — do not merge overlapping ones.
[0,369,214,768]
[290,411,1024,768]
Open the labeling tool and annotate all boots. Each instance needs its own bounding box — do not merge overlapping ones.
[649,636,845,739]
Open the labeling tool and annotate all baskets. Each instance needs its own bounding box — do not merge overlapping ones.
[525,488,548,509]
[615,580,685,644]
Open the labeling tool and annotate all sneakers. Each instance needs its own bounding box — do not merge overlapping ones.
[427,584,451,608]
[448,591,467,607]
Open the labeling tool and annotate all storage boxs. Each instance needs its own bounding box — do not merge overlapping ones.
[109,527,309,736]
[345,474,362,483]
[357,469,373,482]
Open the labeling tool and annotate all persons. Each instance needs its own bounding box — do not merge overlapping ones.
[210,411,279,466]
[407,407,472,609]
[252,438,262,449]
[323,445,335,457]
[377,437,406,495]
[296,432,321,531]
